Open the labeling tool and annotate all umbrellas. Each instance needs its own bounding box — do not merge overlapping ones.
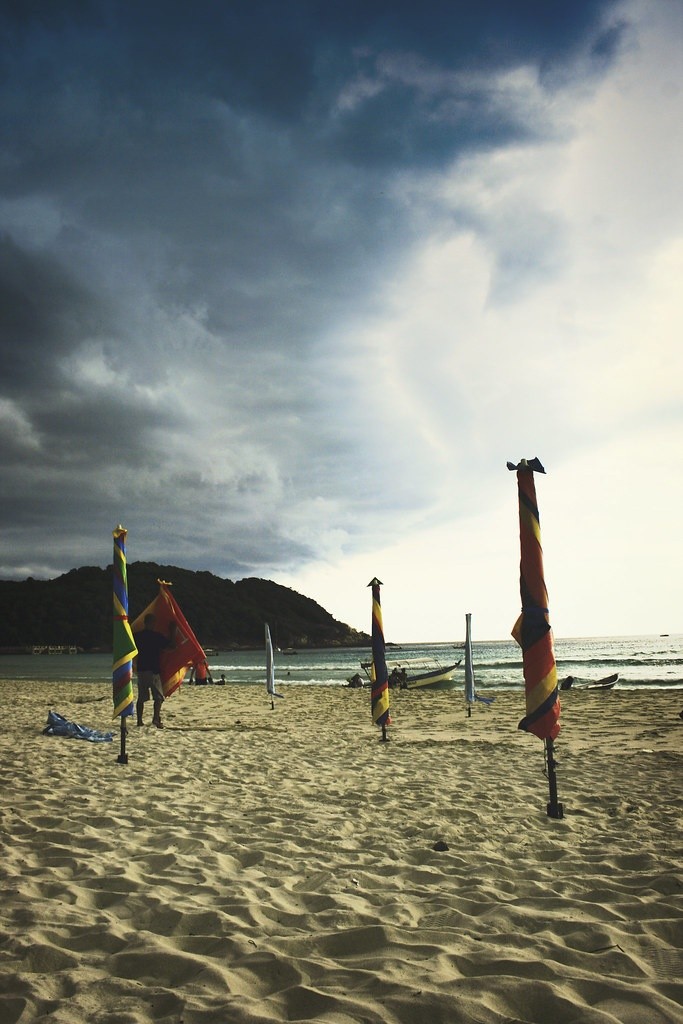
[131,582,207,700]
[368,578,390,741]
[264,625,276,703]
[508,457,563,822]
[464,613,473,716]
[113,526,138,764]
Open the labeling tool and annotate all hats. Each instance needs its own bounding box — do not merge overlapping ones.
[144,614,155,625]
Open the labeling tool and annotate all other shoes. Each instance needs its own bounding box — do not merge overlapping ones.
[152,716,166,729]
[137,720,143,725]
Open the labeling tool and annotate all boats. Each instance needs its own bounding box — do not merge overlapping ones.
[202,648,218,656]
[560,671,620,690]
[389,644,401,650]
[452,644,466,649]
[283,647,298,654]
[358,657,462,689]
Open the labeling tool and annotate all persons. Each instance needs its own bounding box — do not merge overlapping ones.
[187,658,212,684]
[388,668,407,690]
[351,673,364,687]
[560,676,573,690]
[215,674,225,685]
[132,614,176,728]
